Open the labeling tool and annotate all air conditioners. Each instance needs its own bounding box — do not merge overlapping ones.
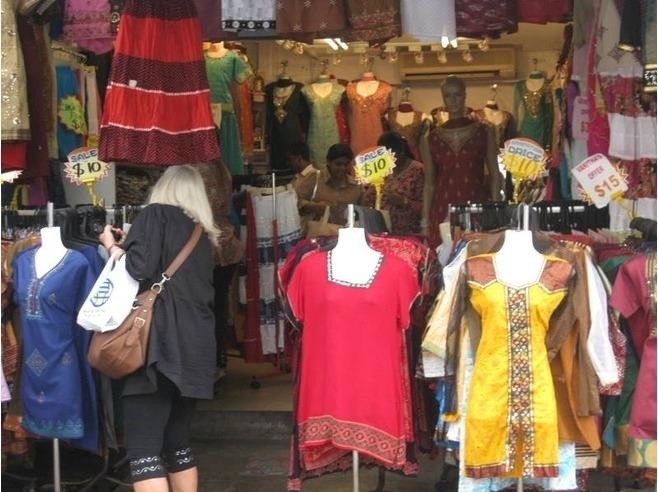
[398,48,518,82]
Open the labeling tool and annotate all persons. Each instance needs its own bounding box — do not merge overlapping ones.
[288,225,420,470]
[98,165,222,492]
[285,140,320,200]
[297,143,377,225]
[376,130,425,235]
[12,226,92,441]
[420,72,500,255]
[461,230,582,480]
[201,38,557,173]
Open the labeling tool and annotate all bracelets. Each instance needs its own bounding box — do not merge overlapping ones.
[108,243,118,257]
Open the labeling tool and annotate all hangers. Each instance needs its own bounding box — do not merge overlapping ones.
[1,202,141,245]
[320,204,390,252]
[444,199,589,231]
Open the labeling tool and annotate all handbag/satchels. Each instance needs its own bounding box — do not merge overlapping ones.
[86,290,157,379]
